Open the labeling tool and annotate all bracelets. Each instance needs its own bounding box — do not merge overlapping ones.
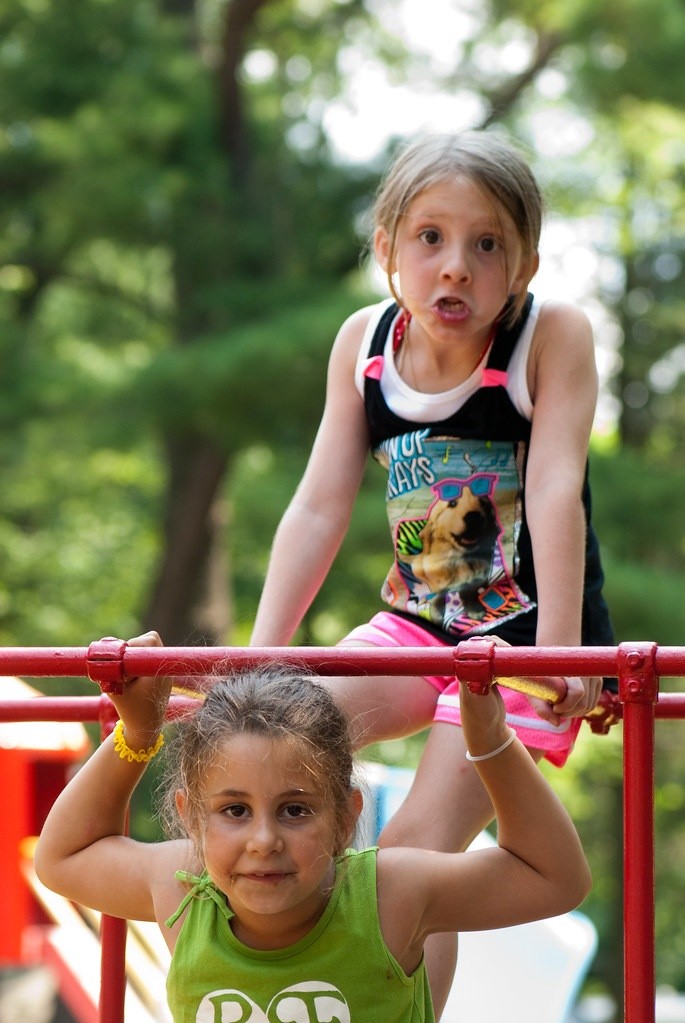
[113,720,165,763]
[466,728,516,761]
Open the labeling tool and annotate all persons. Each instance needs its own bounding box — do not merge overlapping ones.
[35,631,591,1023]
[249,130,617,1023]
